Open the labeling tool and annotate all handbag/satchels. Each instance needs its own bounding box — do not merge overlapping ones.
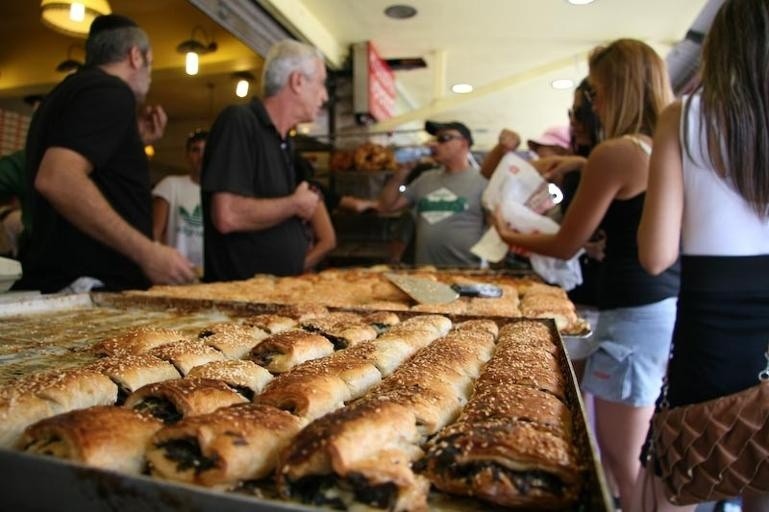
[649,379,769,507]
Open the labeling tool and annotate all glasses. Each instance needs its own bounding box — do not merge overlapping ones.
[568,109,577,120]
[584,88,598,102]
[189,147,202,152]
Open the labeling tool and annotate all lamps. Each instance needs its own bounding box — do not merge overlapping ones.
[39,0,112,40]
[176,25,217,76]
[231,71,257,97]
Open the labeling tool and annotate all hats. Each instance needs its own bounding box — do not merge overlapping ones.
[89,15,138,34]
[425,121,472,146]
[527,127,569,152]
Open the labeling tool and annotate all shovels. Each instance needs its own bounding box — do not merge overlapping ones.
[383,273,503,305]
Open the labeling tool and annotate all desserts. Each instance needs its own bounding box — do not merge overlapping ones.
[0,264,586,512]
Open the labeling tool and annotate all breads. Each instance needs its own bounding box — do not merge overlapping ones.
[335,142,396,171]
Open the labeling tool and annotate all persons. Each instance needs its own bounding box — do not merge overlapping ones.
[492,38,682,511]
[302,157,379,269]
[201,39,329,284]
[379,123,487,268]
[0,149,42,290]
[633,1,769,512]
[152,130,210,282]
[488,75,609,389]
[27,15,195,291]
